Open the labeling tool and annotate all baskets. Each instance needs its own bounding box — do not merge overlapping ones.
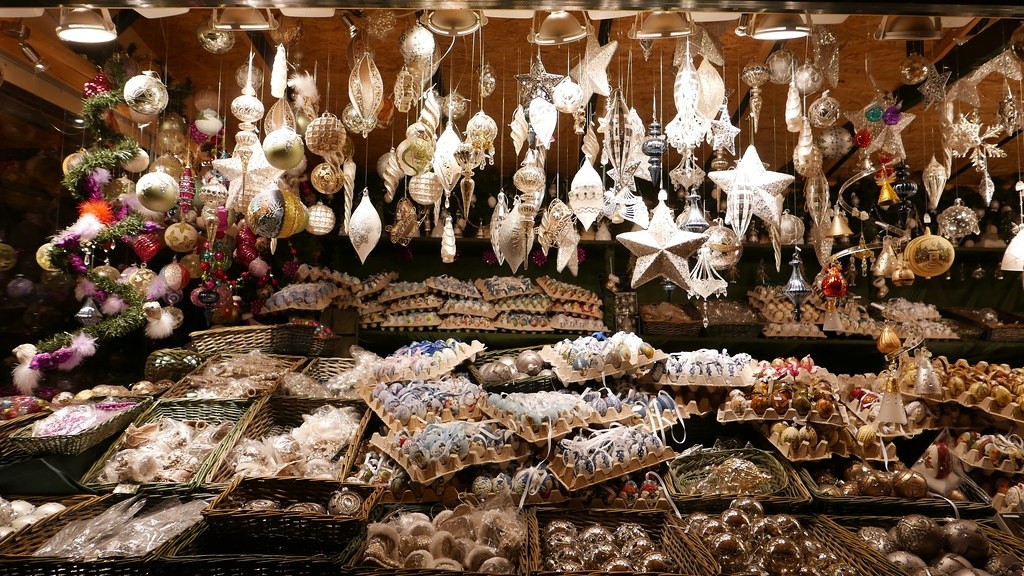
[1,304,1024,576]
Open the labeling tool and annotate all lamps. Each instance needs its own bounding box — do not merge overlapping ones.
[424,9,488,37]
[628,11,700,39]
[211,9,279,31]
[873,15,943,42]
[527,11,593,46]
[53,7,117,44]
[748,13,813,41]
